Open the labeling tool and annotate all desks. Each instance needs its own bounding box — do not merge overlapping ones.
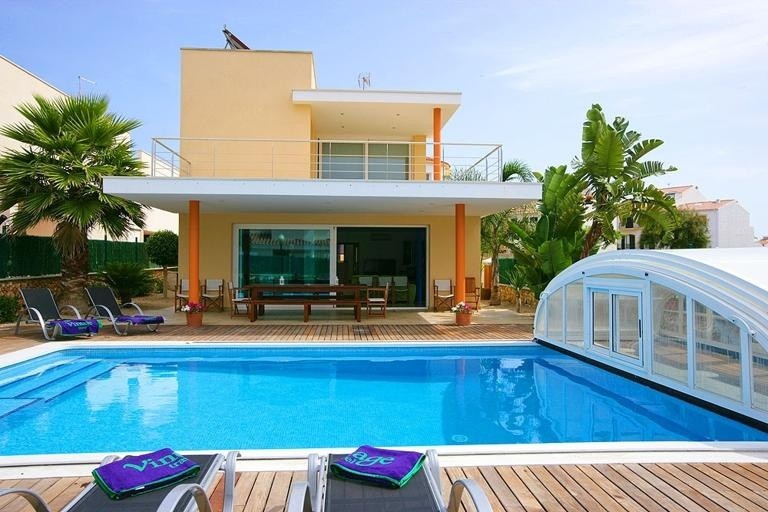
[243,283,366,322]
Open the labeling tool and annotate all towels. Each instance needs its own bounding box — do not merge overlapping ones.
[91,448,200,501]
[329,440,428,489]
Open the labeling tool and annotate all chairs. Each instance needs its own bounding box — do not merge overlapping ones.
[429,275,484,312]
[284,448,494,511]
[0,451,245,512]
[174,280,254,320]
[366,280,392,315]
[14,280,164,343]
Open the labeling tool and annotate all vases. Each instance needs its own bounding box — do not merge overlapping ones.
[457,312,472,325]
[186,311,202,326]
